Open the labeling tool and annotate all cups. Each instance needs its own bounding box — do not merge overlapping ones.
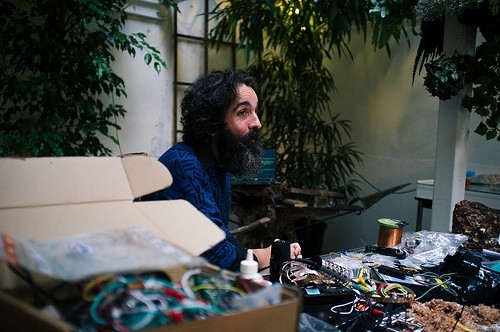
[378,225,403,248]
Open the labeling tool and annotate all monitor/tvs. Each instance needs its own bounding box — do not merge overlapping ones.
[230,147,276,186]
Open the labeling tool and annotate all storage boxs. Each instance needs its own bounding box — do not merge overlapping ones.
[1,151,303,332]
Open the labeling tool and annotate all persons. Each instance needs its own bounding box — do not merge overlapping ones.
[134,67,301,273]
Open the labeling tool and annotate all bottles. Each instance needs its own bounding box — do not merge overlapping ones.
[314,174,328,207]
[240,249,259,275]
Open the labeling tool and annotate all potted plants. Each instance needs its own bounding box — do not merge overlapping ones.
[194,0,423,258]
